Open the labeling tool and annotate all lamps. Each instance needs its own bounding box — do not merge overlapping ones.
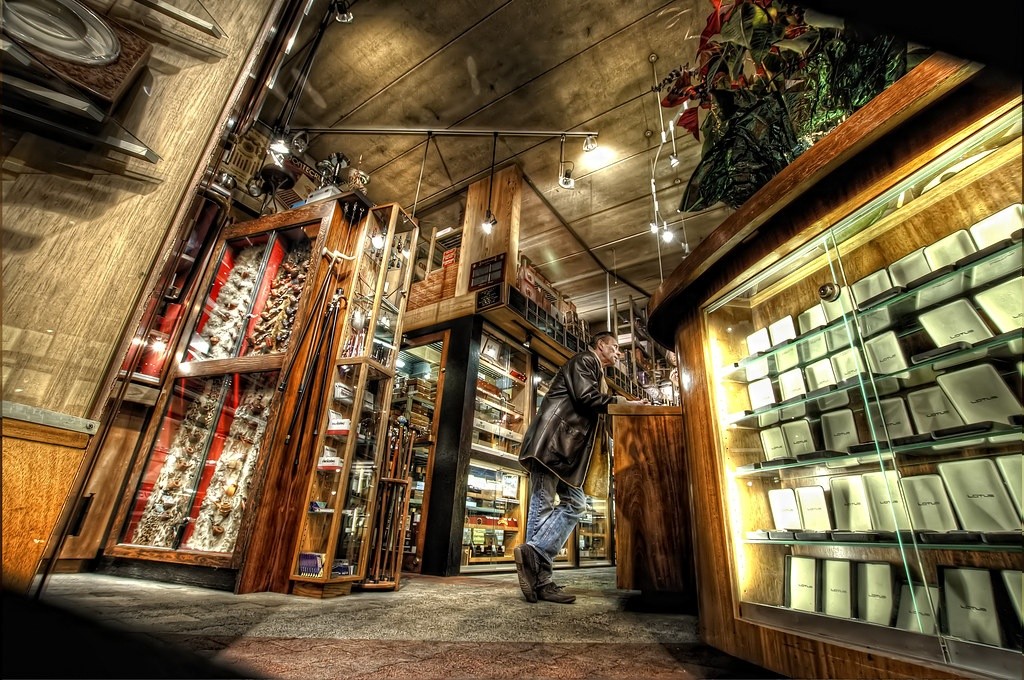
[668,120,679,168]
[482,132,497,234]
[582,135,598,153]
[335,0,354,23]
[559,133,574,188]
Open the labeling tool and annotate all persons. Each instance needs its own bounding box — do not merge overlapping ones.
[514,332,650,603]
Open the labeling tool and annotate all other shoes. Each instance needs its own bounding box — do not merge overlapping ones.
[540,582,584,600]
[512,544,542,599]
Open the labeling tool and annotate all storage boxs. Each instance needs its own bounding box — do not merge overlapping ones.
[405,247,460,309]
[513,250,654,390]
[304,186,340,204]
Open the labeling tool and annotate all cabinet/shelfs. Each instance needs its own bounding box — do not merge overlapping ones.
[369,315,533,577]
[607,403,697,594]
[529,354,614,569]
[612,295,655,385]
[709,103,1024,680]
[99,200,369,591]
[288,202,420,600]
